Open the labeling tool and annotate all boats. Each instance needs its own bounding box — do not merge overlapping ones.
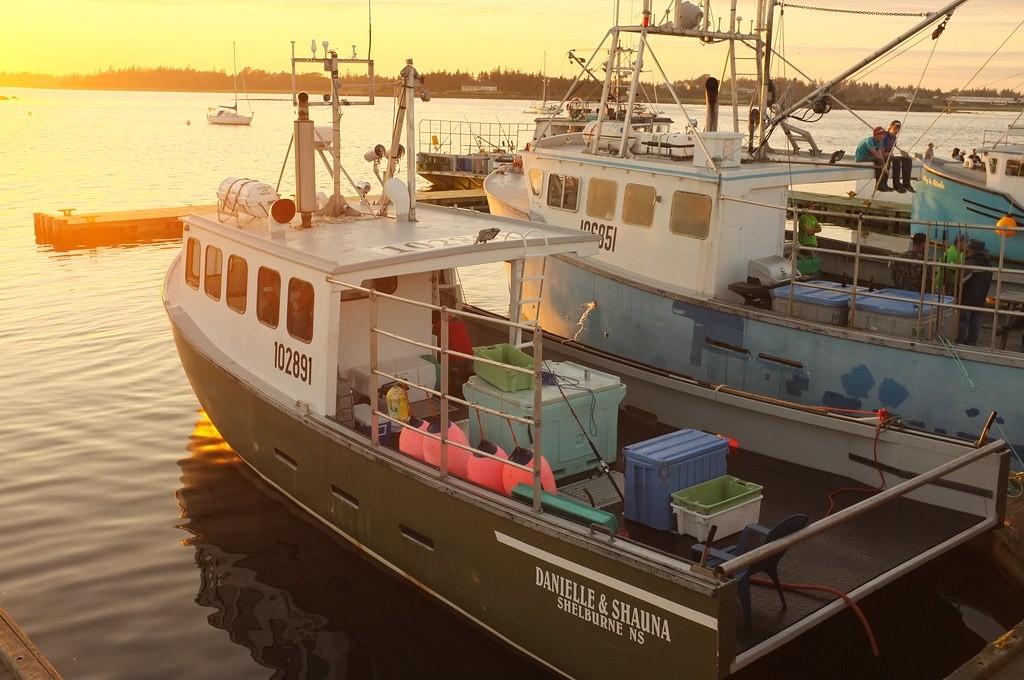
[415,0,672,190]
[483,0,1024,463]
[162,0,1009,680]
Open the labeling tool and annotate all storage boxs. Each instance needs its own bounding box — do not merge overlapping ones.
[797,255,824,274]
[693,132,745,168]
[769,280,876,326]
[347,343,763,543]
[848,288,958,344]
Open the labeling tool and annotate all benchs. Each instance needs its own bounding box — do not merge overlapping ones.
[980,323,1024,334]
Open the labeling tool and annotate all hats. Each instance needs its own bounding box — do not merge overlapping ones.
[968,239,985,250]
[802,201,816,208]
[890,120,901,127]
[960,150,966,154]
[873,126,885,136]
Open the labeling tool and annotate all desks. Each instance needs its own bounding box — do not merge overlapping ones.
[992,291,1024,350]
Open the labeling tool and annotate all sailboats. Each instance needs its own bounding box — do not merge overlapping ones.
[523,50,562,115]
[206,42,254,125]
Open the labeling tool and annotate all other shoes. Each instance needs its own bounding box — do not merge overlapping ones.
[875,185,893,192]
[893,183,907,193]
[903,184,916,193]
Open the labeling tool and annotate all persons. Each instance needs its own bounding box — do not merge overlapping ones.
[924,143,993,173]
[955,239,995,344]
[429,309,473,397]
[855,128,893,192]
[890,233,936,295]
[884,120,917,194]
[936,235,971,296]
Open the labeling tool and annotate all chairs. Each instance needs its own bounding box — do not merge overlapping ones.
[691,513,810,627]
